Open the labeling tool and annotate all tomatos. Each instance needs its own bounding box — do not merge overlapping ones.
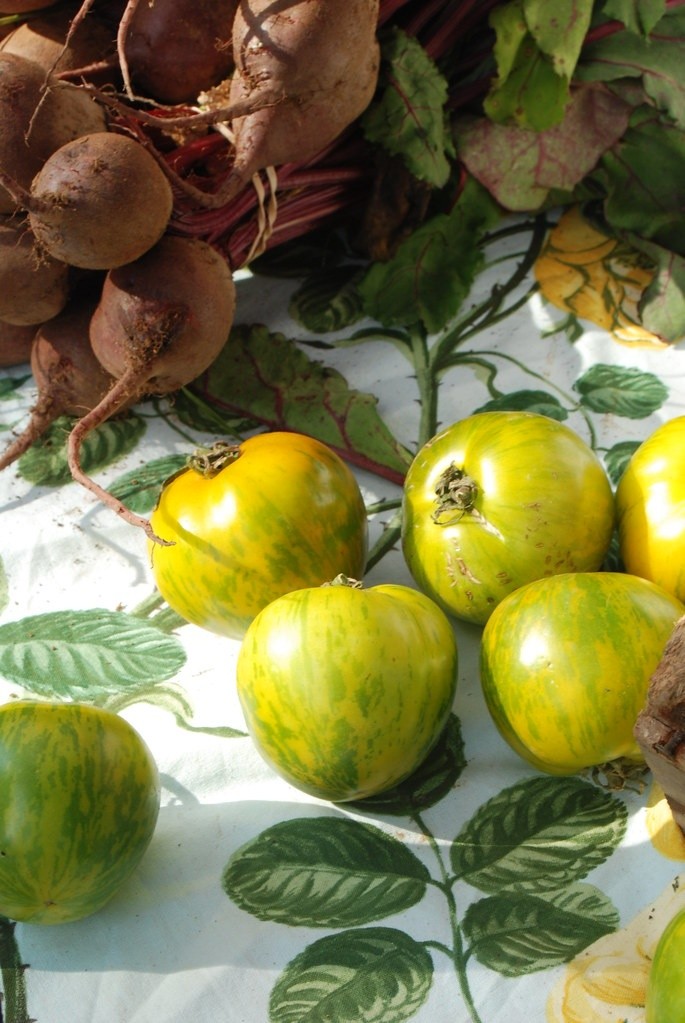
[0,700,160,922]
[149,412,684,806]
